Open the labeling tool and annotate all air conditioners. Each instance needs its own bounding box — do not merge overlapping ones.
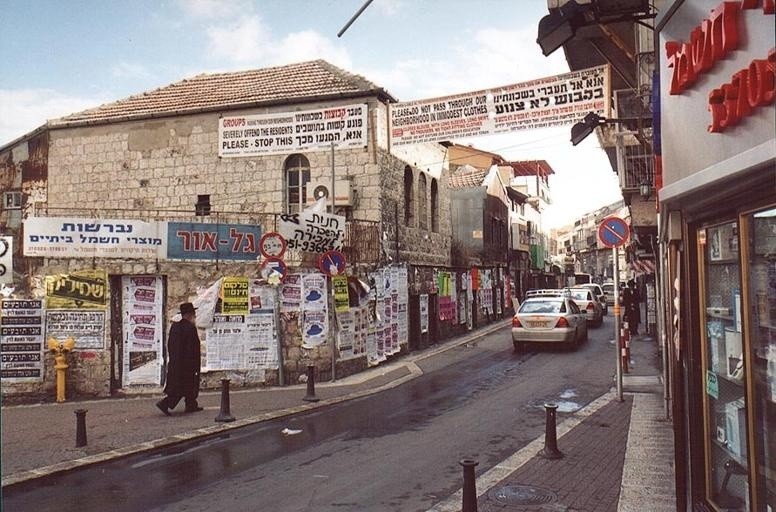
[305,180,357,208]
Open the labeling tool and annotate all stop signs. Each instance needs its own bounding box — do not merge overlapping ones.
[260,258,287,283]
[319,251,346,277]
[598,217,630,246]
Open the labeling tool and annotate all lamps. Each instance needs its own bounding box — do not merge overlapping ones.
[536,0,659,58]
[638,177,655,202]
[569,112,654,147]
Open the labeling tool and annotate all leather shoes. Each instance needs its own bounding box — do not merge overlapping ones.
[184,406,204,413]
[155,401,171,416]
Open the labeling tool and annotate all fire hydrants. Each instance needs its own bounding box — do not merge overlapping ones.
[48,337,75,402]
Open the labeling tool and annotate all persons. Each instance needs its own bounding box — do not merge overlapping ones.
[156,302,204,416]
[622,280,642,336]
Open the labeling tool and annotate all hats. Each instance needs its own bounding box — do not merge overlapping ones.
[306,324,323,336]
[305,290,322,301]
[177,303,199,315]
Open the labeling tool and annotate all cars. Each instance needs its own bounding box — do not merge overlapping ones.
[511,283,624,353]
